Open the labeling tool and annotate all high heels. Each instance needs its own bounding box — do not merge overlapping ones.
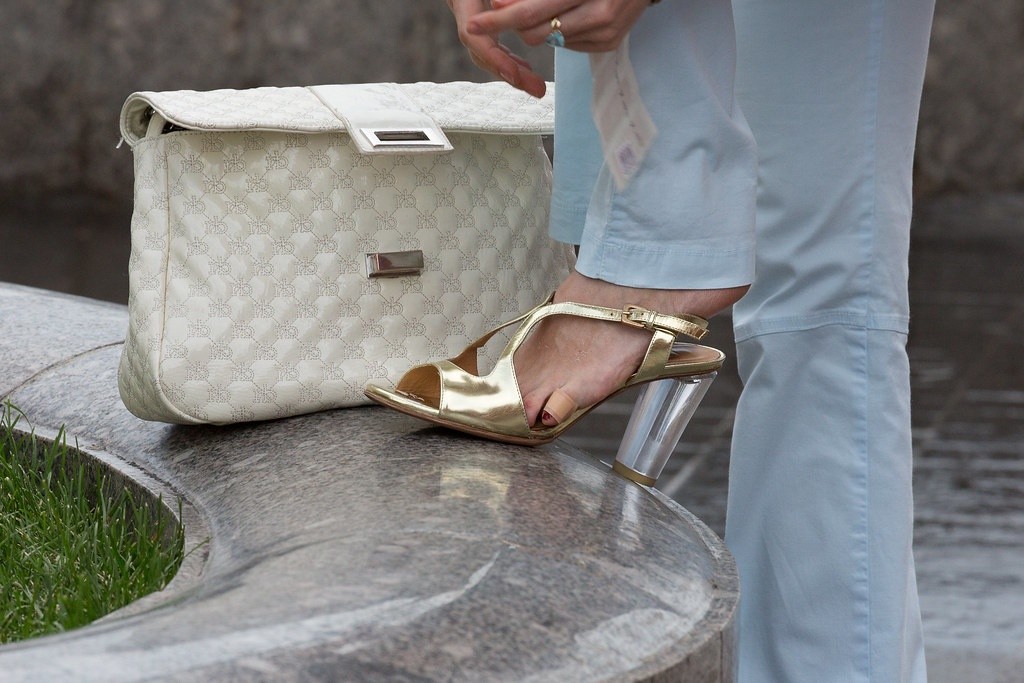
[364,289,726,488]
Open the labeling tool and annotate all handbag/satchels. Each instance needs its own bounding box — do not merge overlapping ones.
[118,81,560,425]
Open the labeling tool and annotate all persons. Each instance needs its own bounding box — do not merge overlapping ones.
[364,0,937,683]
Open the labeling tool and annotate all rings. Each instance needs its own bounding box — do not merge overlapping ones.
[546,17,564,46]
